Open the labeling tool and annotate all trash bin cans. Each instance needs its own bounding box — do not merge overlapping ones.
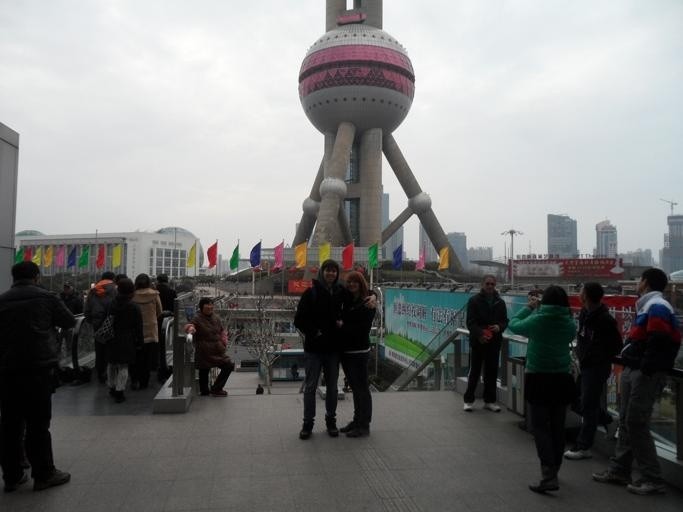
[506,356,527,416]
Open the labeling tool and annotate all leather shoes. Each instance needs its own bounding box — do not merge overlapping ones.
[31,471,70,490]
[6,470,27,491]
[340,422,368,437]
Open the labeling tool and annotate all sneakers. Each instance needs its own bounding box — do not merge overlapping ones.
[529,479,561,494]
[604,414,620,439]
[626,480,667,496]
[484,402,500,412]
[325,418,339,436]
[463,398,476,411]
[108,387,126,403]
[210,386,227,396]
[566,449,593,460]
[300,418,314,439]
[593,470,632,485]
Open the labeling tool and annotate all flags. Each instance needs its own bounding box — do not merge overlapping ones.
[11,240,451,274]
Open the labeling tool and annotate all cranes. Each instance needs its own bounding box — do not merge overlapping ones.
[659,197,678,216]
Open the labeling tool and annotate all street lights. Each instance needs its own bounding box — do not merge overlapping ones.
[376,286,384,344]
[500,228,524,290]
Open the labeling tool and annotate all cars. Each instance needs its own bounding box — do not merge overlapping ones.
[241,335,272,346]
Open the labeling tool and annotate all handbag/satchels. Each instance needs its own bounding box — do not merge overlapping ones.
[95,313,116,346]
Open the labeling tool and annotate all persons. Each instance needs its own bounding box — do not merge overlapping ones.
[335,266,378,438]
[592,266,680,495]
[508,285,577,492]
[463,275,510,411]
[0,261,76,492]
[60,270,179,404]
[184,297,233,397]
[564,282,625,458]
[293,258,378,440]
